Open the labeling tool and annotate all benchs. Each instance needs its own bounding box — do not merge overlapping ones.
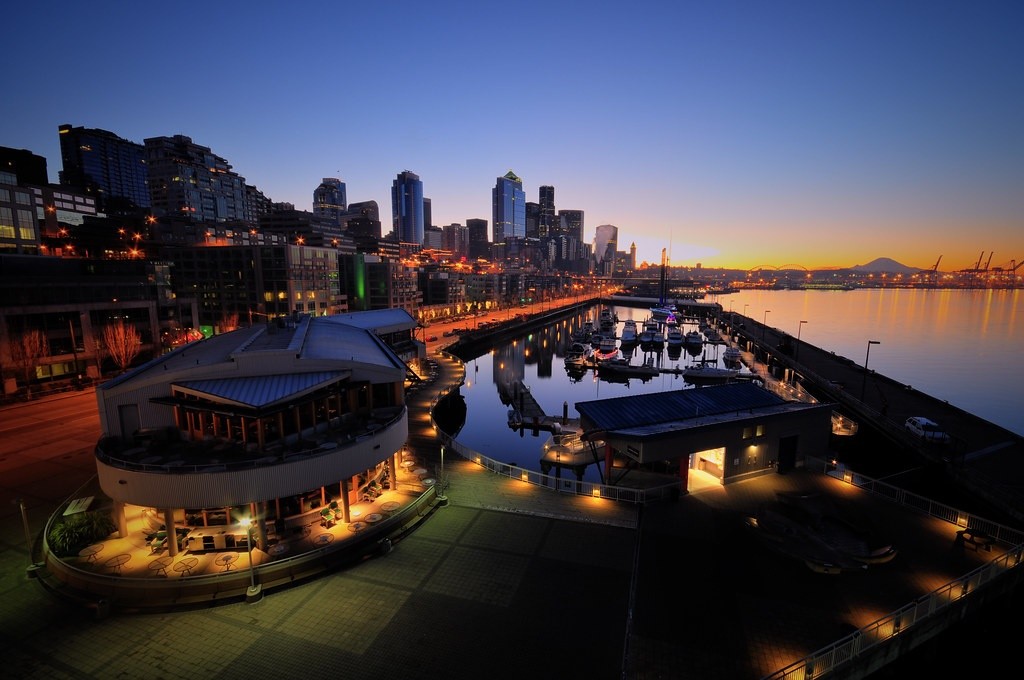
[954,530,996,553]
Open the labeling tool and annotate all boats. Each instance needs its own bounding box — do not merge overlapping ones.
[726,345,743,364]
[563,299,720,383]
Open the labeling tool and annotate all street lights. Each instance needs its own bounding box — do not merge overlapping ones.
[729,299,735,313]
[796,320,807,362]
[743,304,749,322]
[762,311,771,342]
[860,339,881,403]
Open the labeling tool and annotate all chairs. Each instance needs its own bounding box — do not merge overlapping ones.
[362,486,375,502]
[320,508,335,528]
[150,530,168,553]
[176,530,188,550]
[329,500,343,523]
[273,518,286,540]
[369,480,382,496]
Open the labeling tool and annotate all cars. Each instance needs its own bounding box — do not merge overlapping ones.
[906,416,952,445]
[423,310,527,343]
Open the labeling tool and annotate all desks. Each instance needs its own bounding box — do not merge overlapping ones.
[148,556,174,578]
[79,543,104,563]
[313,533,334,549]
[381,501,400,517]
[964,528,988,541]
[173,557,199,577]
[268,544,290,561]
[399,450,436,485]
[347,520,366,534]
[364,513,382,527]
[215,551,239,572]
[104,554,131,574]
[123,412,396,471]
